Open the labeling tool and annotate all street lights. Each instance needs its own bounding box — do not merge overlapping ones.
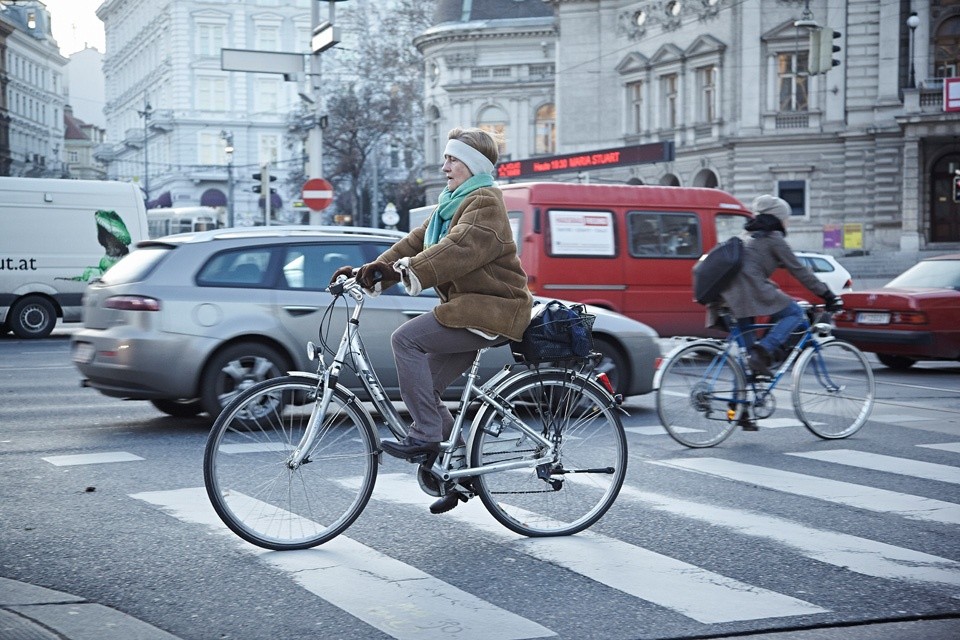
[138,102,152,211]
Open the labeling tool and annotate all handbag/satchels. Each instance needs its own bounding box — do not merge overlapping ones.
[694,230,768,306]
[523,300,590,360]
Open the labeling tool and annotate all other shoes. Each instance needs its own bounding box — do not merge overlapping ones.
[750,357,774,378]
[728,418,758,431]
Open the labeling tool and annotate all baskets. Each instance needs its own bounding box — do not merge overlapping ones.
[523,313,596,364]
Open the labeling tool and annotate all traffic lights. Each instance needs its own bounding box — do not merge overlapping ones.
[808,27,841,74]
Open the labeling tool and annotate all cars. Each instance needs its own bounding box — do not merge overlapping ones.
[792,249,854,294]
[829,253,960,369]
[70,224,665,431]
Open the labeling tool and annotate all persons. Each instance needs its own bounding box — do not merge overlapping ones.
[330,128,532,514]
[706,194,842,430]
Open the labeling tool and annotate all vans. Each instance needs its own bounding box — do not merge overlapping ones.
[502,180,825,359]
[0,175,150,338]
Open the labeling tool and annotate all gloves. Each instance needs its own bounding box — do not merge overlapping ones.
[356,261,407,286]
[825,295,843,312]
[330,266,352,284]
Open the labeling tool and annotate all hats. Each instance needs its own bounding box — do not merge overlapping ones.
[755,195,792,219]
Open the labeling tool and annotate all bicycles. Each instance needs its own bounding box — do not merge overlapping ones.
[652,304,875,448]
[203,275,632,552]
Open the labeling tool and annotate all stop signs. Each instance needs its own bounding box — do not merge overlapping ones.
[302,179,333,211]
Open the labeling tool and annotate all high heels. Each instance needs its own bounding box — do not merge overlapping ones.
[430,481,472,514]
[381,435,441,471]
